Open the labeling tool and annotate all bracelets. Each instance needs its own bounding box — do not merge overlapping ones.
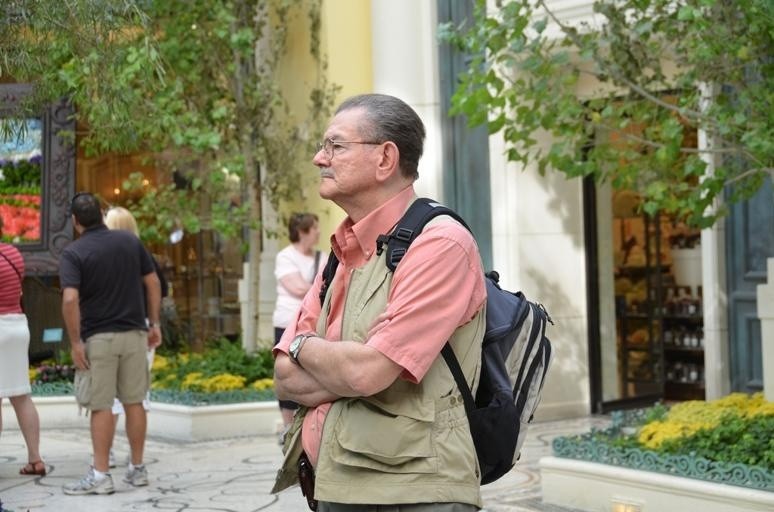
[148,322,161,329]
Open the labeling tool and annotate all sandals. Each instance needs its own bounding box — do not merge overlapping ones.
[20,462,46,474]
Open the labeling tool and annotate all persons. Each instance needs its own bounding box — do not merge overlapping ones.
[55,189,163,497]
[0,211,50,474]
[86,205,172,469]
[268,94,490,511]
[270,212,331,441]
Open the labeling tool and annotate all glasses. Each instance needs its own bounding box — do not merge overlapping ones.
[317,138,382,160]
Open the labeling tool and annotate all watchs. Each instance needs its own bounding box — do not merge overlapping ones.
[288,332,316,371]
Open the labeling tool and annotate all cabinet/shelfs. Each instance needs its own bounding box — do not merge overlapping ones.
[620,305,705,401]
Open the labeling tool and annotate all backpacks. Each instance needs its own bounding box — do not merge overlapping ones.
[319,197,552,485]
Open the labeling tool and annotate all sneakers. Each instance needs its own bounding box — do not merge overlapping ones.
[121,464,149,486]
[63,470,113,495]
[90,452,117,468]
